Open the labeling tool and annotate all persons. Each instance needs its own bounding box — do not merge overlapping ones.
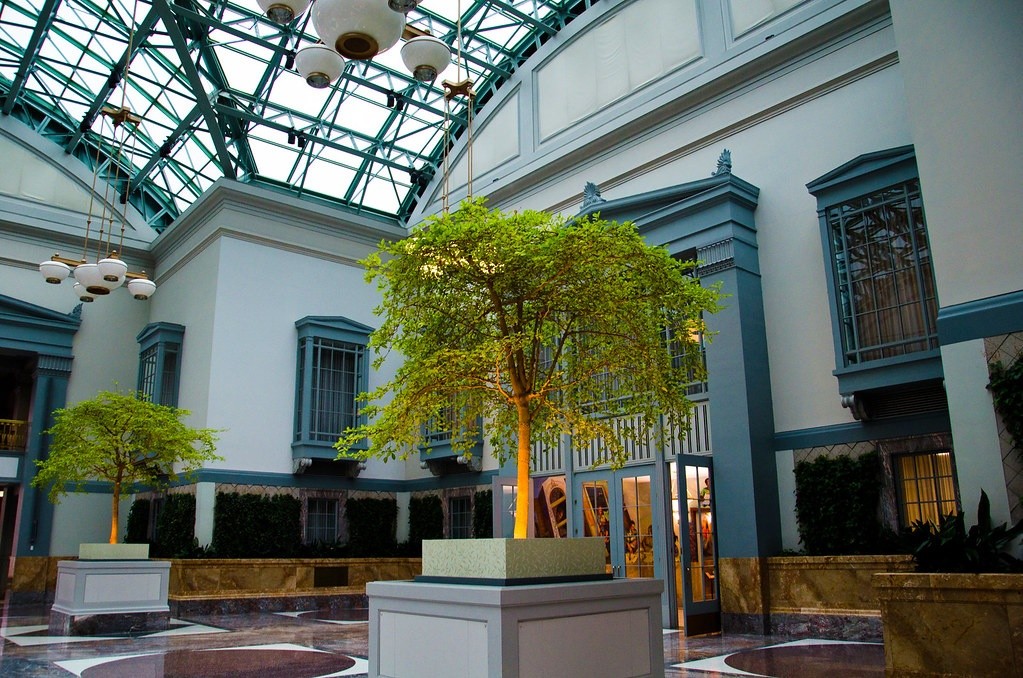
[550,506,680,562]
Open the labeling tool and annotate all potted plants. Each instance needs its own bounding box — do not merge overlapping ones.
[333,197,736,677]
[30,379,232,637]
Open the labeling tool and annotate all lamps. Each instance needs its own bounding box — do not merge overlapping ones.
[288,126,296,144]
[406,0,505,277]
[108,66,121,89]
[80,115,91,133]
[296,130,306,148]
[39,1,156,302]
[285,51,294,70]
[385,89,394,108]
[409,167,417,184]
[418,172,425,186]
[255,0,452,88]
[396,93,404,111]
